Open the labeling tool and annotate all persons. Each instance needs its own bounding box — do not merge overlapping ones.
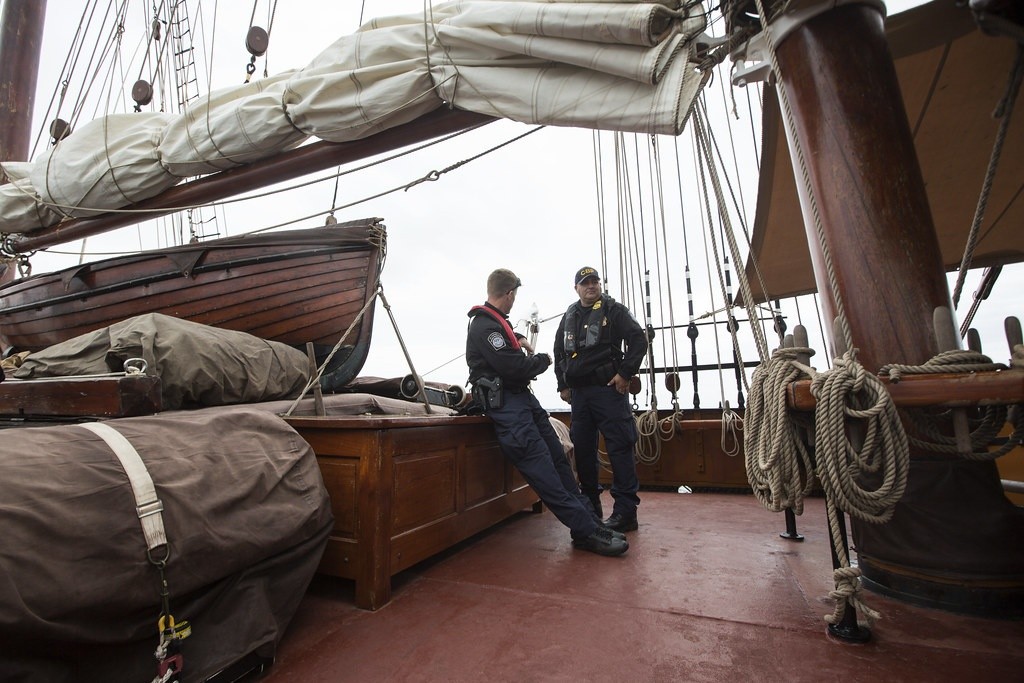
[466,268,629,557]
[553,267,648,533]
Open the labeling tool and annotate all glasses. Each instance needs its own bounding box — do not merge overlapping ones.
[506,278,521,294]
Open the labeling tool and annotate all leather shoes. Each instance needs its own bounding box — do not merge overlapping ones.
[596,524,627,541]
[601,506,639,531]
[575,526,630,556]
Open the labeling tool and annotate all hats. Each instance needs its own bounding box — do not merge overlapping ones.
[575,267,600,285]
